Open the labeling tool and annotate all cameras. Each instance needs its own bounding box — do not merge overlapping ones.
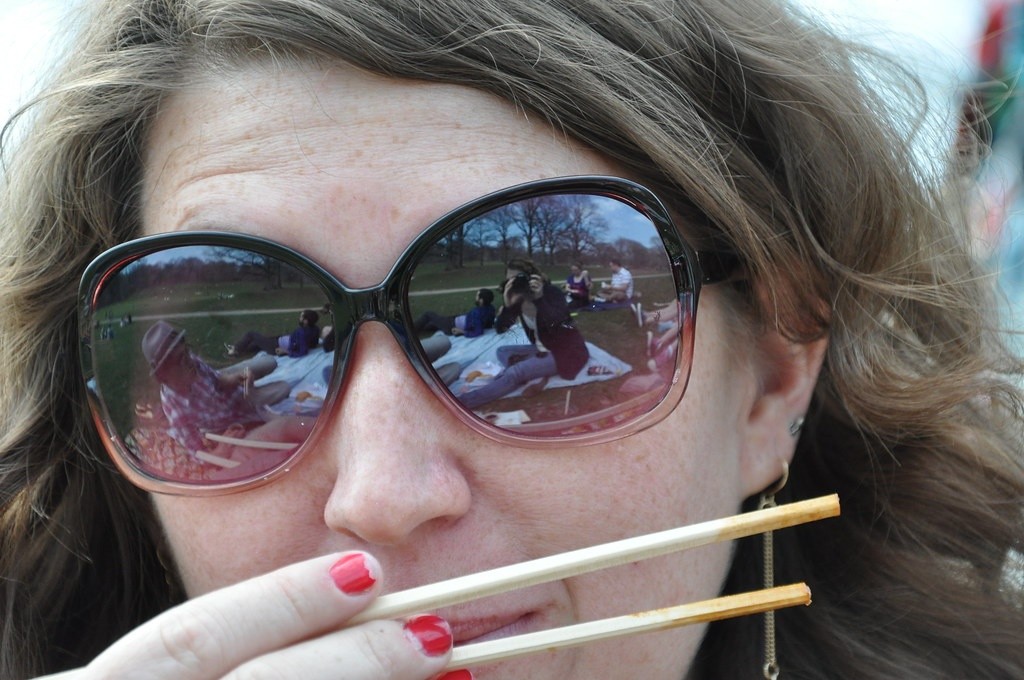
[511,273,531,293]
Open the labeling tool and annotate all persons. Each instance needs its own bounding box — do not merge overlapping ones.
[0,0,1023,680]
[413,257,680,413]
[90,308,335,470]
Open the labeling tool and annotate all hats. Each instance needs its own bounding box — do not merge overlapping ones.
[142,320,187,377]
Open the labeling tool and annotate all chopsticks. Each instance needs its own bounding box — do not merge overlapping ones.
[331,494,840,671]
[195,433,299,468]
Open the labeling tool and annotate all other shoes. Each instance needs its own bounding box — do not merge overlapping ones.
[644,330,662,357]
[630,301,648,328]
[522,374,548,399]
[647,347,671,372]
[224,341,235,356]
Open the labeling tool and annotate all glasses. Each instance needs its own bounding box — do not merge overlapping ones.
[79,172,755,496]
[160,343,189,373]
